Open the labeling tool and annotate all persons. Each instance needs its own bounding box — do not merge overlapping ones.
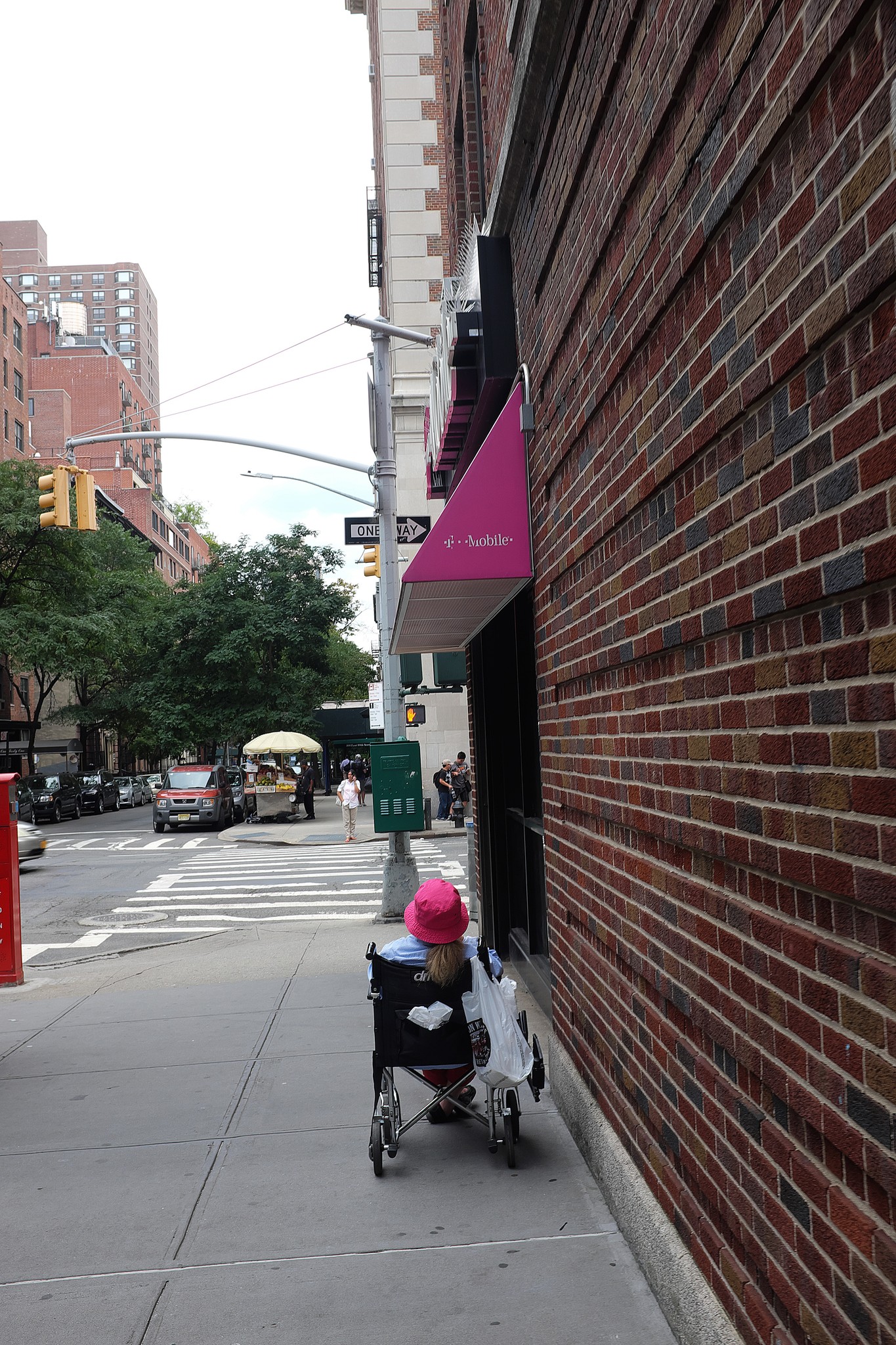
[340,754,372,807]
[447,751,472,821]
[337,769,361,842]
[367,879,504,1124]
[301,760,315,820]
[433,759,458,821]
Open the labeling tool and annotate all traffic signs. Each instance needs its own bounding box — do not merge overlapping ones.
[343,515,432,545]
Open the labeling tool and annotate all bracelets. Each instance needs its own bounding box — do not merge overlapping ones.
[354,783,357,785]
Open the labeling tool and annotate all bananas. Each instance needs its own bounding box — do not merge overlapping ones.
[276,783,295,790]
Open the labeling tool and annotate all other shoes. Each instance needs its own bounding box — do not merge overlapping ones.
[435,817,447,821]
[345,838,350,842]
[350,837,357,840]
[306,816,315,820]
[426,1093,453,1124]
[362,803,367,806]
[463,814,468,818]
[303,816,309,820]
[453,1085,476,1114]
[358,804,362,807]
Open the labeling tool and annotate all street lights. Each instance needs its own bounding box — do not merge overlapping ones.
[241,468,423,925]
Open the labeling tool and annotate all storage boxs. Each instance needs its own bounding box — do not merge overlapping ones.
[244,782,256,794]
[290,762,295,766]
[276,780,298,791]
[290,756,296,762]
[246,772,256,783]
[276,788,295,793]
[247,763,258,772]
[257,774,276,782]
[255,785,276,793]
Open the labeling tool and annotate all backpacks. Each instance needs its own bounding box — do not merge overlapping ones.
[433,770,446,789]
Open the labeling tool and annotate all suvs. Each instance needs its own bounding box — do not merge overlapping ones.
[22,771,84,824]
[72,769,120,816]
[224,758,276,824]
[152,764,234,833]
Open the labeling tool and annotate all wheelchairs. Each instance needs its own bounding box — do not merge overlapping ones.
[365,940,530,1178]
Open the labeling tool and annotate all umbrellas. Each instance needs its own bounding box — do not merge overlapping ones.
[243,731,323,773]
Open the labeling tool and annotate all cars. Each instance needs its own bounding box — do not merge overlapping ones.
[13,779,47,862]
[113,773,165,808]
[287,760,334,781]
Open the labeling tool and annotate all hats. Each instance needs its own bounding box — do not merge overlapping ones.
[442,759,454,765]
[355,754,361,759]
[404,879,469,943]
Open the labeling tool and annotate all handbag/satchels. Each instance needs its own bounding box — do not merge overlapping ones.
[462,955,534,1088]
[335,780,346,806]
[343,760,351,772]
[465,780,472,792]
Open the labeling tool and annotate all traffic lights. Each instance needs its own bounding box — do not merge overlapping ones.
[362,545,381,577]
[405,705,426,724]
[39,468,71,529]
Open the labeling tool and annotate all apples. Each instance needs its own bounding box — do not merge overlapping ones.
[258,766,274,775]
